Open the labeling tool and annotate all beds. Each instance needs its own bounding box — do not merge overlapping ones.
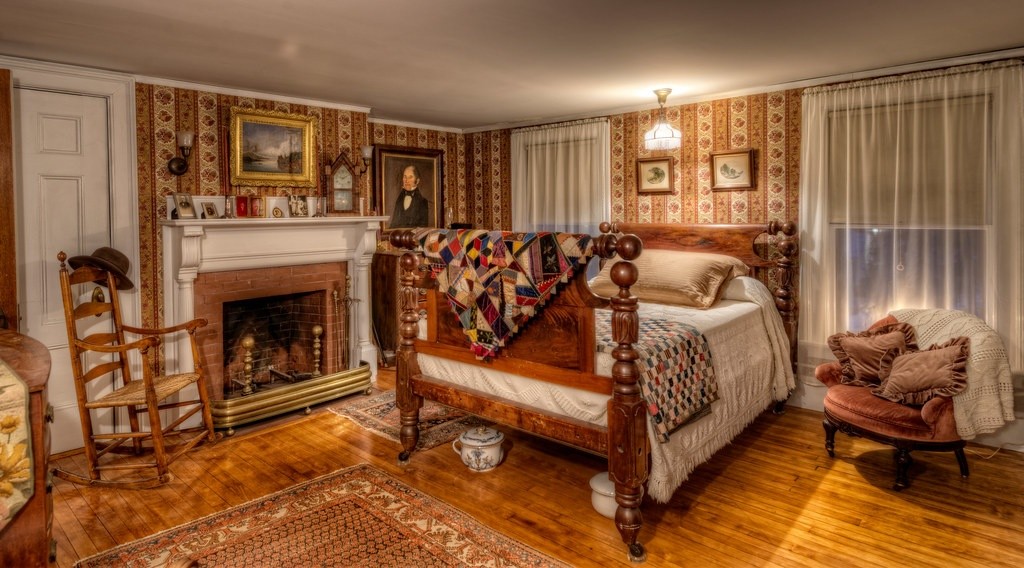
[391,221,802,558]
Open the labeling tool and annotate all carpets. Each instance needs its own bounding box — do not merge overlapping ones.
[592,245,732,309]
[80,458,580,568]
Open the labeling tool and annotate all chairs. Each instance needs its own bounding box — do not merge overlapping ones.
[48,251,222,487]
[812,305,1017,492]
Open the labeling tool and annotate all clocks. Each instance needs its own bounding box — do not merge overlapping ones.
[328,151,358,214]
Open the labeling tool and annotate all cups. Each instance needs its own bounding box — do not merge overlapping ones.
[452,425,505,472]
[589,472,620,520]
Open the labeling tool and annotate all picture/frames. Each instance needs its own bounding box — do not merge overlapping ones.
[229,104,318,191]
[368,143,445,237]
[635,156,676,195]
[201,201,220,219]
[173,193,197,220]
[709,148,756,193]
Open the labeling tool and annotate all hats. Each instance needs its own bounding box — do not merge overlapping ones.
[67,246,134,290]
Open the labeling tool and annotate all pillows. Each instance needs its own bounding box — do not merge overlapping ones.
[827,320,922,388]
[711,252,753,301]
[871,338,971,405]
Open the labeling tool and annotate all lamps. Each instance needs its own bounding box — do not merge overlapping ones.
[358,143,374,174]
[645,89,682,150]
[167,128,196,175]
